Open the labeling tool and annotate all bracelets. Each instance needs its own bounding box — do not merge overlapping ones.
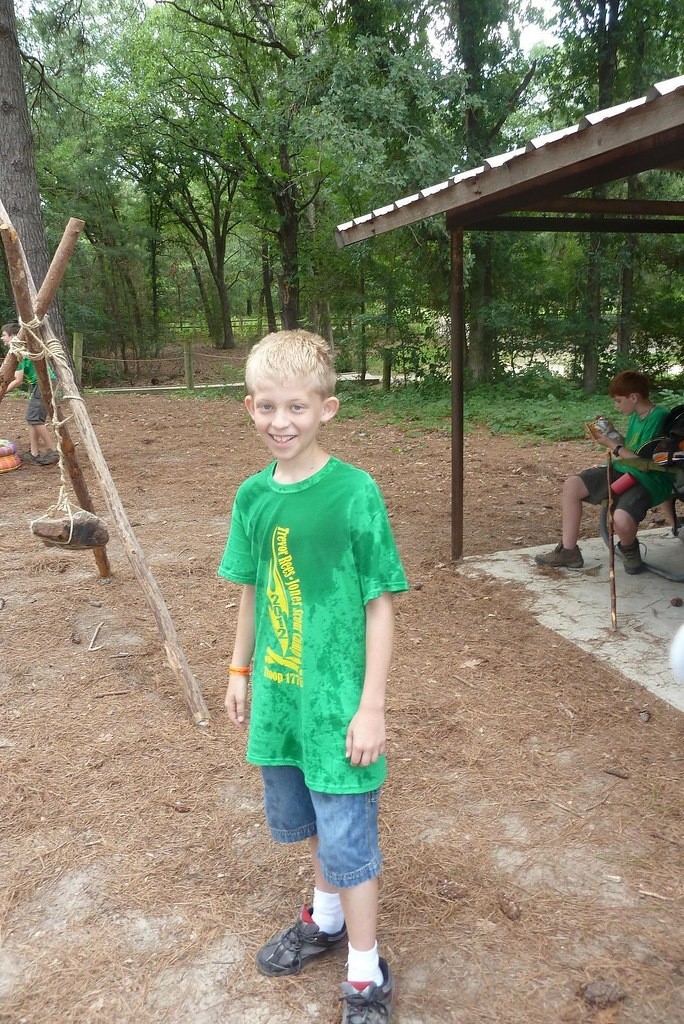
[226,665,252,679]
[612,444,623,457]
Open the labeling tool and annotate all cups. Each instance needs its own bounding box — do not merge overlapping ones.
[610,472,636,496]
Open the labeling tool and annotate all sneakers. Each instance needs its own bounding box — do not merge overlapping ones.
[36,449,61,464]
[534,540,584,572]
[338,956,396,1024]
[255,903,348,978]
[614,536,648,575]
[17,447,44,466]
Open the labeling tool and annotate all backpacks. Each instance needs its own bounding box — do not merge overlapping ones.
[664,404,684,440]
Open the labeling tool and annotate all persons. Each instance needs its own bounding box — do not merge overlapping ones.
[205,329,411,1024]
[534,370,669,575]
[1,323,58,464]
